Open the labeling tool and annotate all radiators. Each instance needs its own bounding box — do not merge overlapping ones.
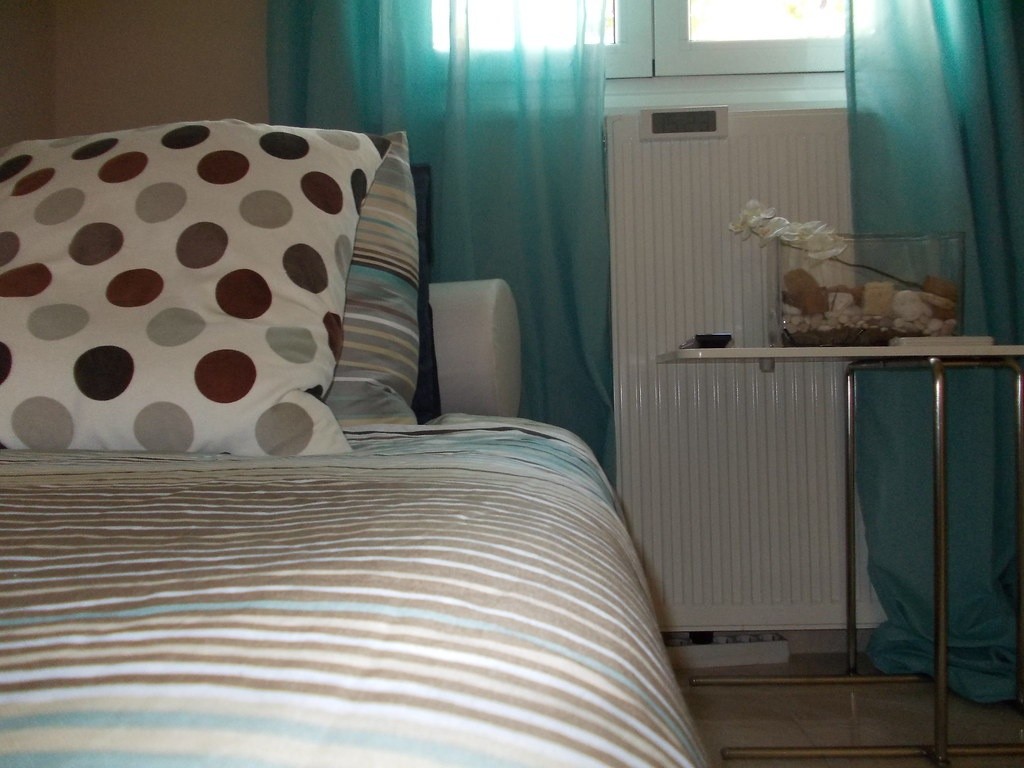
[605,106,872,605]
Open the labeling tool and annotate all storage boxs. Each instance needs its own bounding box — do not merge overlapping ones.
[774,233,965,346]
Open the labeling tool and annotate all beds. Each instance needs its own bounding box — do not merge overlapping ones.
[0,279,711,768]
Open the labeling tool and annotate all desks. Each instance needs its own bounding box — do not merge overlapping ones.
[657,336,1023,768]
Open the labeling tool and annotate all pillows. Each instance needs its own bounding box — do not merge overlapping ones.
[322,131,419,424]
[0,118,392,457]
